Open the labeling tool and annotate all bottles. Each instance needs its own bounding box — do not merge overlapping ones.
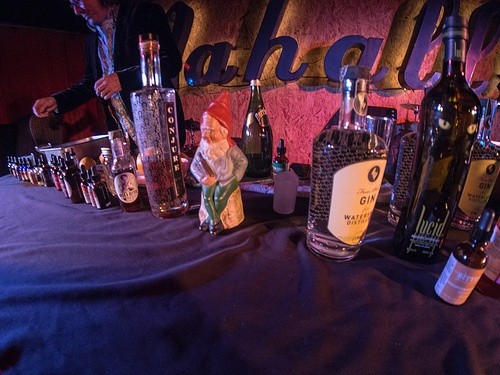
[476,215,500,299]
[111,137,142,212]
[242,79,273,178]
[7,147,112,209]
[130,33,190,219]
[272,139,290,186]
[434,209,495,306]
[392,13,482,267]
[450,73,500,231]
[306,65,388,264]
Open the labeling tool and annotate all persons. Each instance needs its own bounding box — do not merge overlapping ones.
[190,102,248,238]
[32,0,187,151]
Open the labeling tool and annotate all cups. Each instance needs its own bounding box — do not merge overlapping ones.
[388,132,418,227]
[365,105,396,147]
[273,171,299,214]
